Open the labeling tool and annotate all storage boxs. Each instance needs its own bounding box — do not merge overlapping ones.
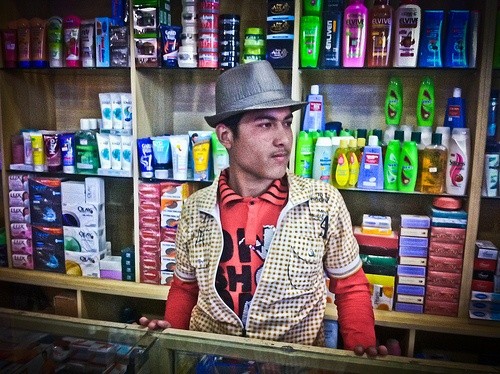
[7,171,134,283]
[351,198,468,317]
[131,0,173,67]
[109,27,131,67]
[469,240,500,322]
[138,180,197,286]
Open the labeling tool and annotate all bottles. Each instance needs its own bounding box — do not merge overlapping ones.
[74,118,101,175]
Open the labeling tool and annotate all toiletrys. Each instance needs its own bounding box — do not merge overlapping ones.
[3,0,129,68]
[137,130,231,181]
[299,0,479,69]
[295,75,471,195]
[481,97,500,199]
[10,92,133,174]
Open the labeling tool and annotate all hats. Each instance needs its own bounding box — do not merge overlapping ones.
[203,60,311,128]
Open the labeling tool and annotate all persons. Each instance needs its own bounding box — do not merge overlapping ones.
[139,60,400,374]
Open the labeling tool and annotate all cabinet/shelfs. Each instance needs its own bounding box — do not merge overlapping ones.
[0,0,500,341]
